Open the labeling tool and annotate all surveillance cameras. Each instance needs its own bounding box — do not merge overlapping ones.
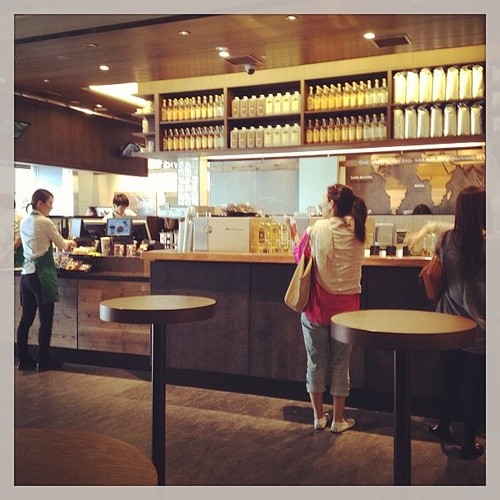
[244,64,255,74]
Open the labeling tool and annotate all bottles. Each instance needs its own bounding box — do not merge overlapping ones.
[142,116,149,133]
[306,113,386,143]
[306,78,388,111]
[247,127,256,148]
[139,241,148,257]
[231,91,299,117]
[239,127,247,148]
[249,213,292,256]
[159,229,178,250]
[256,126,264,148]
[230,126,239,149]
[163,124,224,151]
[273,123,301,146]
[422,231,436,257]
[264,125,273,147]
[161,93,224,122]
[133,236,137,246]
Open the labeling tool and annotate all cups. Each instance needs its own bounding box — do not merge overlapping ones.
[114,243,125,256]
[126,243,136,256]
[141,148,145,153]
[137,109,142,114]
[100,237,111,255]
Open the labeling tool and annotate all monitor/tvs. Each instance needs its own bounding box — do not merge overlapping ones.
[80,218,153,246]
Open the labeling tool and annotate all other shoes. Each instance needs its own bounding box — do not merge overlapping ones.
[461,442,484,461]
[313,412,330,430]
[16,359,32,370]
[428,424,451,440]
[36,359,62,373]
[331,418,355,433]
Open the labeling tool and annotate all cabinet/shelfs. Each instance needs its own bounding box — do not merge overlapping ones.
[130,43,487,162]
[330,309,478,486]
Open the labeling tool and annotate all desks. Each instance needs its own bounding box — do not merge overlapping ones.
[14,427,161,486]
[99,294,217,486]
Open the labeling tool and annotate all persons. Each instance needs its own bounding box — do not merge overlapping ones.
[102,194,129,223]
[404,204,434,253]
[429,186,486,454]
[293,183,368,432]
[87,208,97,216]
[17,189,71,371]
[14,200,24,267]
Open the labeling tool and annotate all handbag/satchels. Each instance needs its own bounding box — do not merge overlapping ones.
[284,225,313,314]
[418,230,448,302]
